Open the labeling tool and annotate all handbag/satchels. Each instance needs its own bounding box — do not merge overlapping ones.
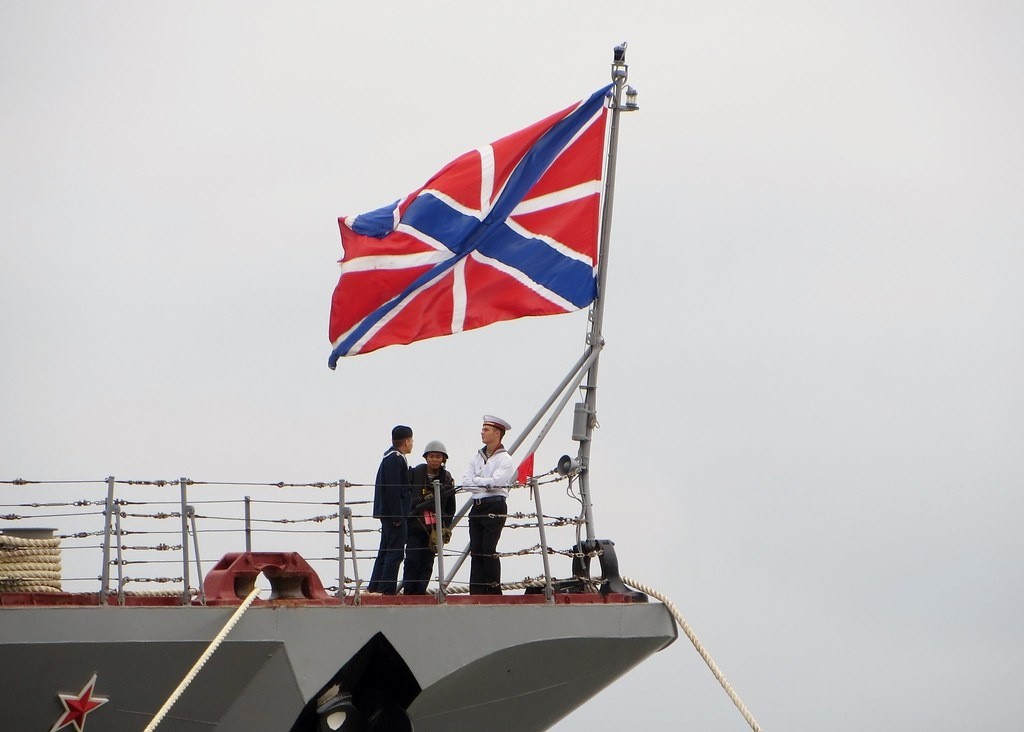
[430,528,444,552]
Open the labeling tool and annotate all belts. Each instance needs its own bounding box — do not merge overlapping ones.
[474,495,506,507]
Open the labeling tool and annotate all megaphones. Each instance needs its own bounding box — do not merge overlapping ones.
[557,455,580,476]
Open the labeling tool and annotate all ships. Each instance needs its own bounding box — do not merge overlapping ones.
[1,39,677,732]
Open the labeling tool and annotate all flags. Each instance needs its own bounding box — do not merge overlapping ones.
[516,452,535,485]
[328,78,618,370]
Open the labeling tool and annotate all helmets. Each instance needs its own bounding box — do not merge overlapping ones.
[423,441,448,461]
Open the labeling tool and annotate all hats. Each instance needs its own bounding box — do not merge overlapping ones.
[391,425,413,440]
[484,414,511,431]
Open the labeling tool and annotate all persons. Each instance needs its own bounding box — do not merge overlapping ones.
[367,426,413,596]
[461,414,515,595]
[403,440,456,594]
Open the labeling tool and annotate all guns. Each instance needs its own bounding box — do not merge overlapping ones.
[408,484,463,515]
